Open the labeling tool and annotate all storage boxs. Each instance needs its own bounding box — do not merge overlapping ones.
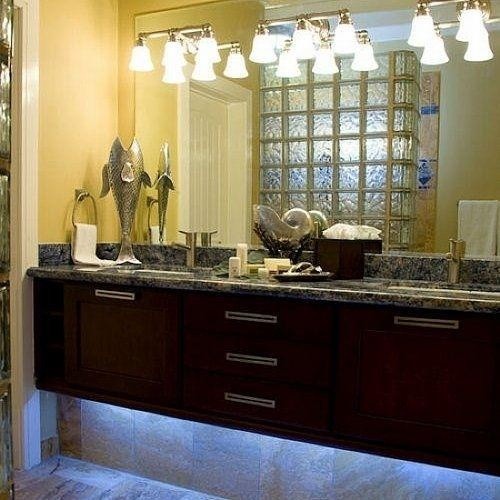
[312,237,364,280]
[361,240,384,256]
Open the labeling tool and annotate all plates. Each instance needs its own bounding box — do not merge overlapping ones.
[274,274,332,284]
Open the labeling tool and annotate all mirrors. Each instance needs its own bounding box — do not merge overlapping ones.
[128,0,500,260]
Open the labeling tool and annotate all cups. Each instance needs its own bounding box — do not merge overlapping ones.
[256,268,269,281]
[229,257,241,278]
[238,243,247,277]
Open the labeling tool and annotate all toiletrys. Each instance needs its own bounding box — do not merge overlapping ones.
[257,268,270,279]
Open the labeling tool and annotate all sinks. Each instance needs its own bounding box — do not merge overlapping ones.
[376,281,500,297]
[117,267,194,276]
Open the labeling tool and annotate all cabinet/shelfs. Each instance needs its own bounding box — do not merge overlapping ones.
[182,279,336,447]
[335,287,500,478]
[32,269,182,415]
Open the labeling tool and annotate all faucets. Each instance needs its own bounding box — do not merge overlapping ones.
[445,238,466,283]
[171,230,198,269]
[201,230,217,247]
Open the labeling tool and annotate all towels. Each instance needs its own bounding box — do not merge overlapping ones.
[359,226,382,242]
[320,223,368,242]
[454,198,499,262]
[69,222,123,268]
[147,226,167,245]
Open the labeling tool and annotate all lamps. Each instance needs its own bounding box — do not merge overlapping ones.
[405,1,500,48]
[275,18,380,79]
[418,0,495,66]
[161,29,250,84]
[128,21,223,73]
[247,7,363,64]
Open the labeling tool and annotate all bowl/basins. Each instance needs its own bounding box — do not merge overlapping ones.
[264,258,292,272]
